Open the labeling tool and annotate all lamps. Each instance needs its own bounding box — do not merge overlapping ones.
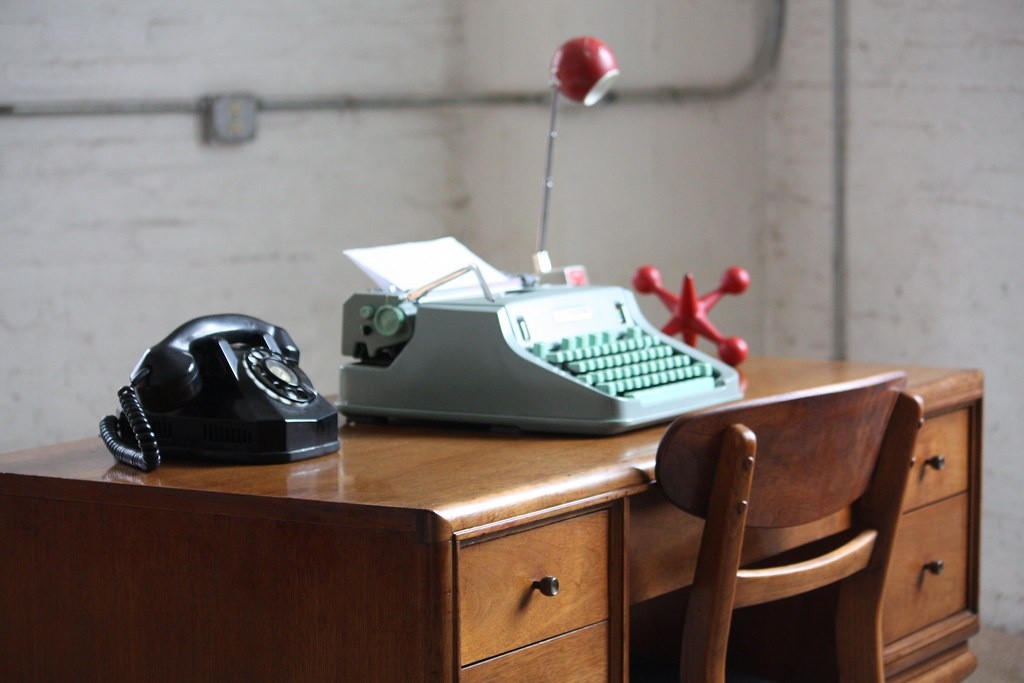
[533,33,620,285]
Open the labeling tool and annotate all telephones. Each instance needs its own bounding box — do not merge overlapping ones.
[118,308,341,463]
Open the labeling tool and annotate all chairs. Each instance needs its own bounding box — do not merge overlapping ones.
[655,371,924,683]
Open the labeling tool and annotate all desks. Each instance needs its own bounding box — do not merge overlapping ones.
[0,362,988,683]
